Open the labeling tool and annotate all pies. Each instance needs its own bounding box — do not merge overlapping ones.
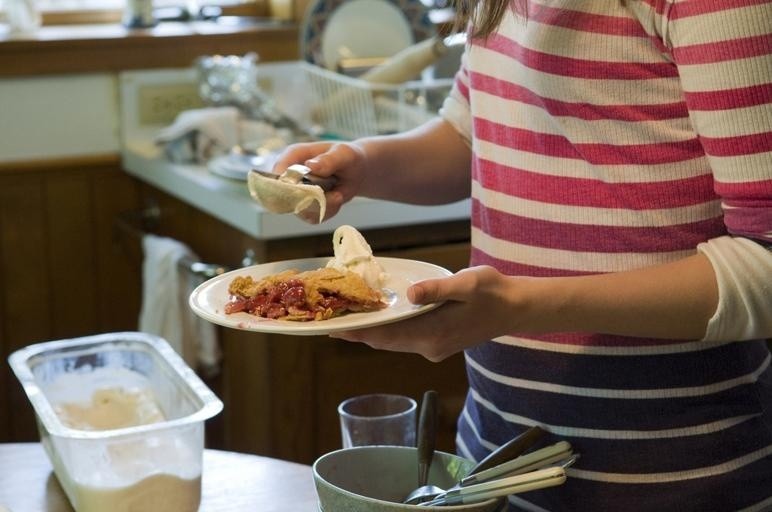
[231,268,388,313]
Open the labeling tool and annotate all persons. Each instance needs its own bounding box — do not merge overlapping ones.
[272,1,771,508]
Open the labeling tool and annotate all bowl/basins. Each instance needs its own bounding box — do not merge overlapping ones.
[312,444,504,512]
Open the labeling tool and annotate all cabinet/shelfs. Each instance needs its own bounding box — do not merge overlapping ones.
[0,165,141,450]
[272,220,472,472]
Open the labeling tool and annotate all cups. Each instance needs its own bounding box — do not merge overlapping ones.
[338,393,416,450]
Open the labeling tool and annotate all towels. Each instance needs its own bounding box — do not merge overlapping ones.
[136,232,224,384]
[153,106,291,166]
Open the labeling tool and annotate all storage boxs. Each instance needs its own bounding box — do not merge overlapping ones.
[7,332,225,512]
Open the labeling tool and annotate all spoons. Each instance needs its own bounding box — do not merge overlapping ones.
[401,439,574,504]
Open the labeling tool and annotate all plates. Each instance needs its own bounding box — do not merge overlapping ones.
[188,254,459,337]
[299,0,439,125]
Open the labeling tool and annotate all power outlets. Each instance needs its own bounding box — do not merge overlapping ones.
[138,85,204,126]
